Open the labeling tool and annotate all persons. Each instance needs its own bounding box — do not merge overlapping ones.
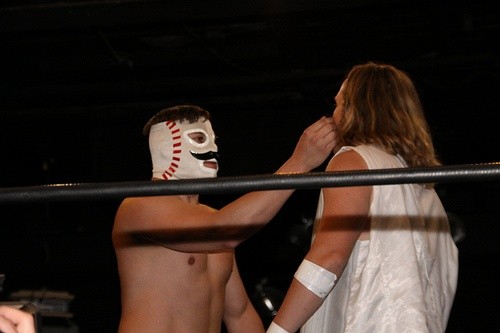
[0,301,42,333]
[112,102,336,333]
[264,62,459,333]
